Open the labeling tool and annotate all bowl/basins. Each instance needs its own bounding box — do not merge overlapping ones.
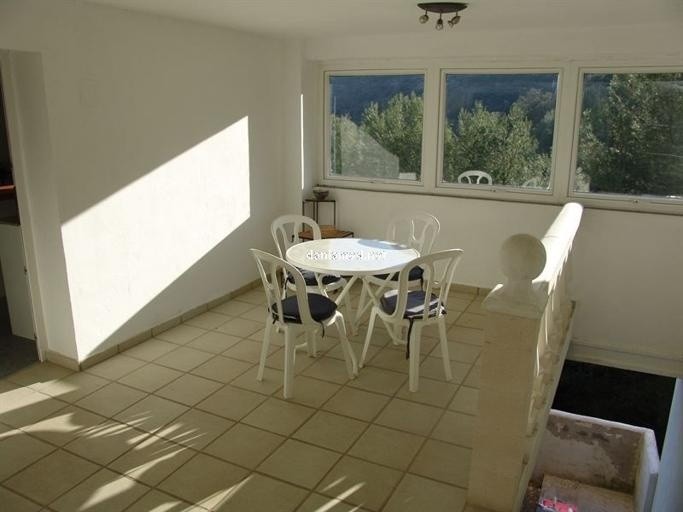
[312,189,329,200]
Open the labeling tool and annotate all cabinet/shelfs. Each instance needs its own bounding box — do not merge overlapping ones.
[296,212,360,239]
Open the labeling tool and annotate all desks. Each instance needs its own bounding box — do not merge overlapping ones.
[291,238,419,357]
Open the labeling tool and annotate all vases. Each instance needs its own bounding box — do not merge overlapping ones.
[311,184,329,201]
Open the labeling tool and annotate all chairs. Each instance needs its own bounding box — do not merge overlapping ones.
[264,213,329,267]
[371,252,461,391]
[366,211,429,291]
[457,171,491,185]
[252,250,359,395]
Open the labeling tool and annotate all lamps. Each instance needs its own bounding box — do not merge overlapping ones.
[416,3,468,31]
